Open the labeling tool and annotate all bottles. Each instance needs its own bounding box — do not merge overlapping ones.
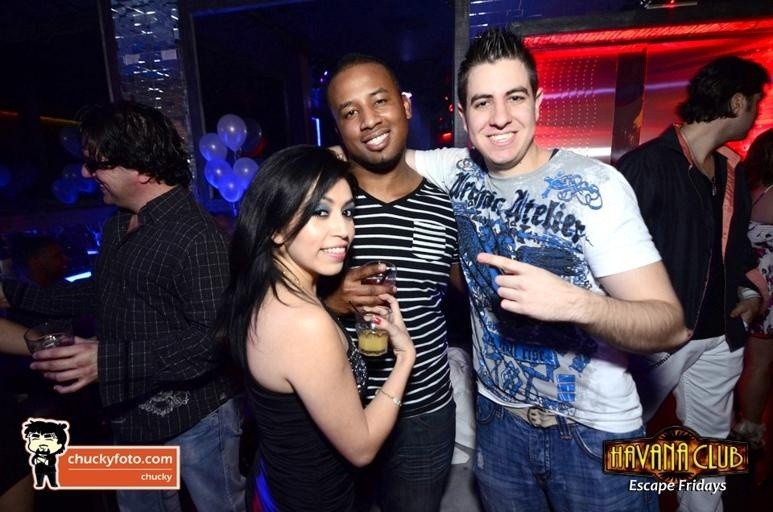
[0,233,15,310]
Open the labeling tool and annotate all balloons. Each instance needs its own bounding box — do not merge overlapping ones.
[199,114,263,203]
[51,164,97,206]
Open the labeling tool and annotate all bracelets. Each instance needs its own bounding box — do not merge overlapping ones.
[378,388,402,407]
[739,288,762,300]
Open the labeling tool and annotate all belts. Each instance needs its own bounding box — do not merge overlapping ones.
[504,406,577,428]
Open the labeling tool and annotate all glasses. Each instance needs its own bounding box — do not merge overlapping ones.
[84,156,119,171]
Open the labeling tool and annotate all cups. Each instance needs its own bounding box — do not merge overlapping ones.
[26,320,75,385]
[364,260,397,288]
[356,311,388,357]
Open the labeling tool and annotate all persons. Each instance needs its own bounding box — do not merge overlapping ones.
[613,55,773,511]
[0,316,49,357]
[0,99,248,512]
[210,142,417,512]
[16,234,73,288]
[730,127,772,424]
[326,26,692,512]
[325,53,470,511]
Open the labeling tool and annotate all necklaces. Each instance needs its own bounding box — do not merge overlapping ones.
[278,265,308,290]
[680,128,718,197]
